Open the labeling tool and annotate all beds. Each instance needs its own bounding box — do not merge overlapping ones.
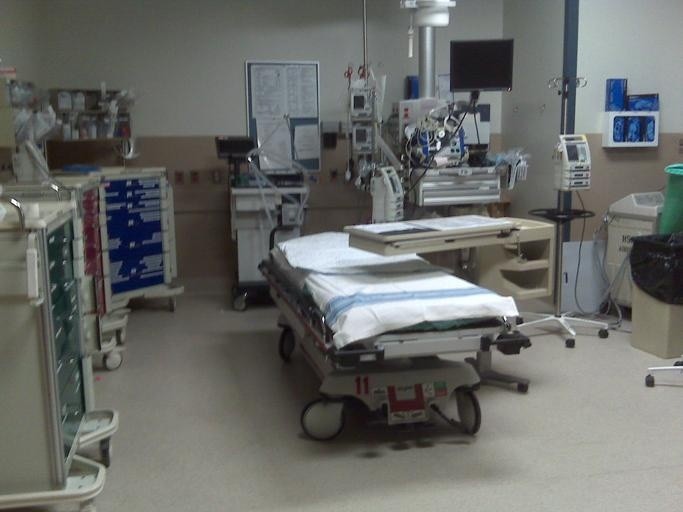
[256,231,529,442]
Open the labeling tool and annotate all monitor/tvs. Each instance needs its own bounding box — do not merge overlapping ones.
[448,38,514,92]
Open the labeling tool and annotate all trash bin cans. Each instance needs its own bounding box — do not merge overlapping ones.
[627,230,683,360]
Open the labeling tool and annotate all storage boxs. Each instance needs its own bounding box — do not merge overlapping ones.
[602,111,659,148]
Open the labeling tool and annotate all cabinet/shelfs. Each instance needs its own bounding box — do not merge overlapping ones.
[0,200,118,511]
[1,168,129,371]
[345,214,554,394]
[57,168,183,312]
